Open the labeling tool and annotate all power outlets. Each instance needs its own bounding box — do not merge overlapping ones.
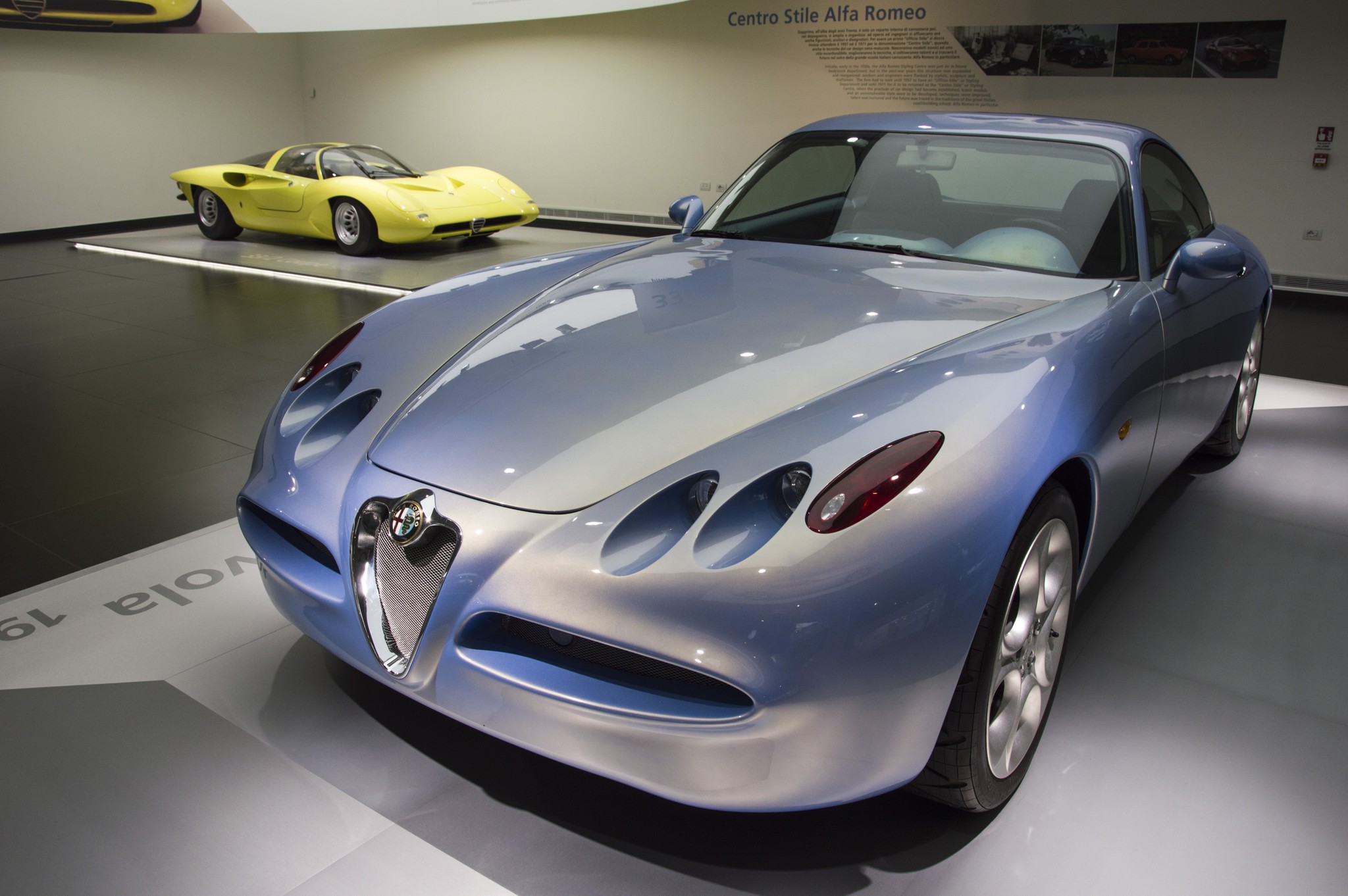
[700,181,710,191]
[716,183,727,193]
[1302,228,1323,241]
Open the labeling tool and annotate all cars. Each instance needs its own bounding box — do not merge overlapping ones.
[1045,32,1273,75]
[169,140,539,258]
[230,109,1276,816]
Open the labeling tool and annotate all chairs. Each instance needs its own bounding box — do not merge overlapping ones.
[846,172,965,250]
[1039,180,1168,278]
